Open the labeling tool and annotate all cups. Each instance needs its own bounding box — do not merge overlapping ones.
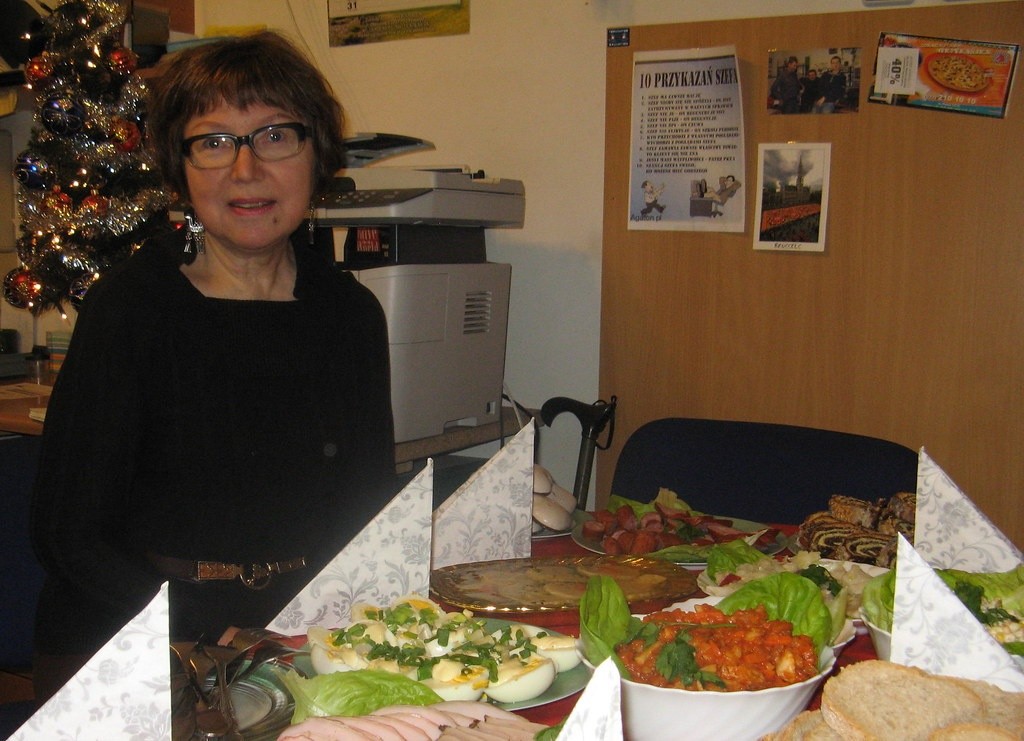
[23,353,52,387]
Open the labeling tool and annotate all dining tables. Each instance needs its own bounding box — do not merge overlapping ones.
[248,510,878,728]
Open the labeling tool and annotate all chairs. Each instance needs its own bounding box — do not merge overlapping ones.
[609,417,917,526]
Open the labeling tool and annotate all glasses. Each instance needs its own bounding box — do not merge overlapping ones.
[181,122,312,169]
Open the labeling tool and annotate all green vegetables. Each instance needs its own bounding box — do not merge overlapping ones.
[278,667,443,722]
[859,563,1023,659]
[577,573,833,690]
[604,491,775,583]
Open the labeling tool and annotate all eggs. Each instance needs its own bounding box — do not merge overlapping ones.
[306,596,581,706]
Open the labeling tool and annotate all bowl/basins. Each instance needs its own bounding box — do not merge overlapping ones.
[575,614,837,741]
[631,595,857,658]
[858,606,1024,674]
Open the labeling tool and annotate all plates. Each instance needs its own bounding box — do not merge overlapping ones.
[695,558,890,613]
[927,55,994,93]
[429,553,700,613]
[294,616,592,712]
[571,515,789,571]
[531,508,593,539]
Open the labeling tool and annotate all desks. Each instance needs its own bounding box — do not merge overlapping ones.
[0,382,53,436]
[392,405,546,476]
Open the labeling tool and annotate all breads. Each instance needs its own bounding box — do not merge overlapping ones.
[753,658,1024,741]
[533,462,578,533]
[796,491,918,567]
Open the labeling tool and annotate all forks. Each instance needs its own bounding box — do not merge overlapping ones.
[190,626,307,741]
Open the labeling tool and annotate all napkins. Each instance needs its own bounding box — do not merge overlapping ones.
[912,446,1024,575]
[428,417,535,571]
[554,655,644,741]
[889,531,1024,693]
[264,458,433,637]
[5,580,172,741]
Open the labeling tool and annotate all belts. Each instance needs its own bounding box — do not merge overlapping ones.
[149,553,306,590]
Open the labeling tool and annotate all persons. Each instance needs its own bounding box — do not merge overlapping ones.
[771,56,846,113]
[29,30,397,709]
